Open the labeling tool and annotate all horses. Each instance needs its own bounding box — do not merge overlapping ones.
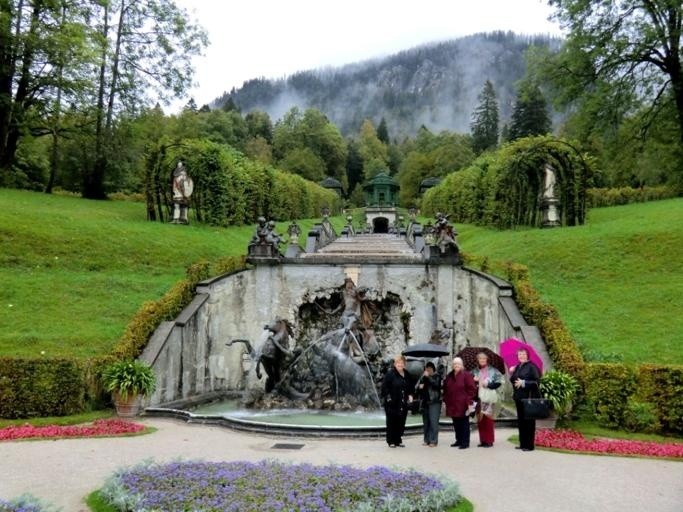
[226,316,294,393]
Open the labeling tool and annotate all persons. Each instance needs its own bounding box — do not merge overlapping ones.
[250,216,287,250]
[381,356,415,448]
[434,211,464,255]
[418,362,441,447]
[508,349,541,451]
[339,287,362,332]
[469,351,506,448]
[442,356,477,449]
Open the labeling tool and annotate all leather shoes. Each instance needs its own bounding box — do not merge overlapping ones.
[477,442,494,447]
[389,442,405,448]
[516,445,534,451]
[422,441,437,447]
[451,441,469,449]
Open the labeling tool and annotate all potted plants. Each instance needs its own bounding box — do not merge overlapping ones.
[538,369,581,418]
[100,354,157,417]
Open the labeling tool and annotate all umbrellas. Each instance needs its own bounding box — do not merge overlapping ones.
[499,336,544,378]
[400,343,450,371]
[456,346,506,376]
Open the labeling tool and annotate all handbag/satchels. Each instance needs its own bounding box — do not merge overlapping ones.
[410,399,423,415]
[479,378,498,402]
[522,399,549,413]
